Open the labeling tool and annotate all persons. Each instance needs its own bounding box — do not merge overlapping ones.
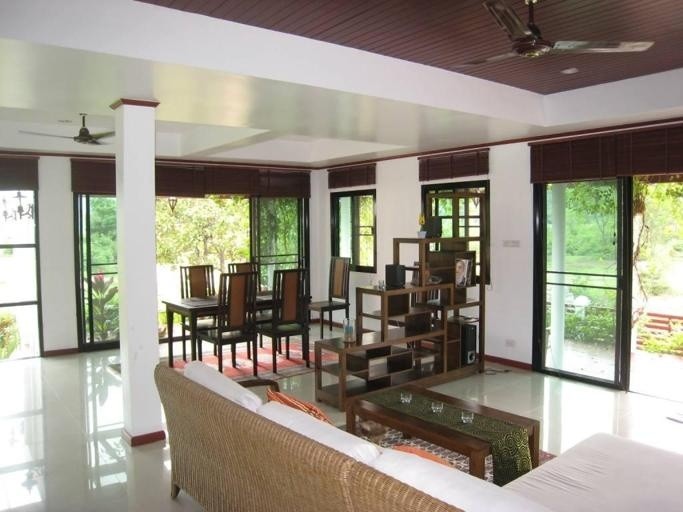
[456,260,466,287]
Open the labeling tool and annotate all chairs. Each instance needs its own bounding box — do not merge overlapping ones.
[158,256,351,376]
[347,431,682,511]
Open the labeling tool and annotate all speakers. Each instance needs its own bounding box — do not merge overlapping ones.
[461,324,476,364]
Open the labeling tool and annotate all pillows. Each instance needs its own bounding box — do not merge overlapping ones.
[268,386,329,428]
[392,444,450,468]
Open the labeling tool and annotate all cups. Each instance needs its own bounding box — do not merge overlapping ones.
[342,318,355,342]
[431,401,443,413]
[461,412,474,423]
[367,275,384,291]
[400,392,412,402]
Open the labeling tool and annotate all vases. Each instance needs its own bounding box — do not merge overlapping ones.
[417,232,426,239]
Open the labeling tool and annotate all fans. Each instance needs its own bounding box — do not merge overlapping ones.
[18,113,115,146]
[453,0,655,70]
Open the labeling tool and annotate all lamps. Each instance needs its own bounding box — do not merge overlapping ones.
[2,191,35,221]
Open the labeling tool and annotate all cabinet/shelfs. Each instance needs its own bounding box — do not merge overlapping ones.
[314,192,485,412]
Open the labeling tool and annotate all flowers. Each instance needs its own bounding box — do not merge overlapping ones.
[418,213,426,231]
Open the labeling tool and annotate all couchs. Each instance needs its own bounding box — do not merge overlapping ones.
[150,362,378,512]
